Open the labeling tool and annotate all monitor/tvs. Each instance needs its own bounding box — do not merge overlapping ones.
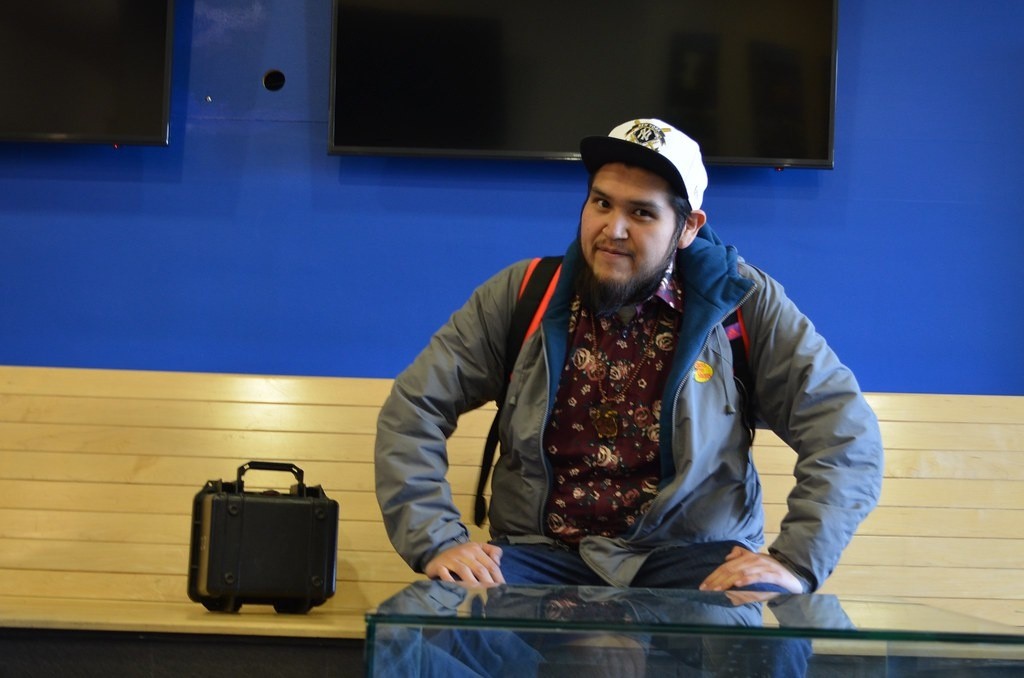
[326,0,840,171]
[0,0,173,147]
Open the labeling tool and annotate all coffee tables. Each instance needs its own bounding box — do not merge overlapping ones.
[364,579,1024,678]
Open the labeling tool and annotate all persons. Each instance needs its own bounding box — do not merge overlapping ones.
[374,119,884,678]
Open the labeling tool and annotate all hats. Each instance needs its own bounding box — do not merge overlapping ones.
[579,118,708,210]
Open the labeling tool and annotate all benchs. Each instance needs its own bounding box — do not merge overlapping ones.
[0,364,1024,678]
